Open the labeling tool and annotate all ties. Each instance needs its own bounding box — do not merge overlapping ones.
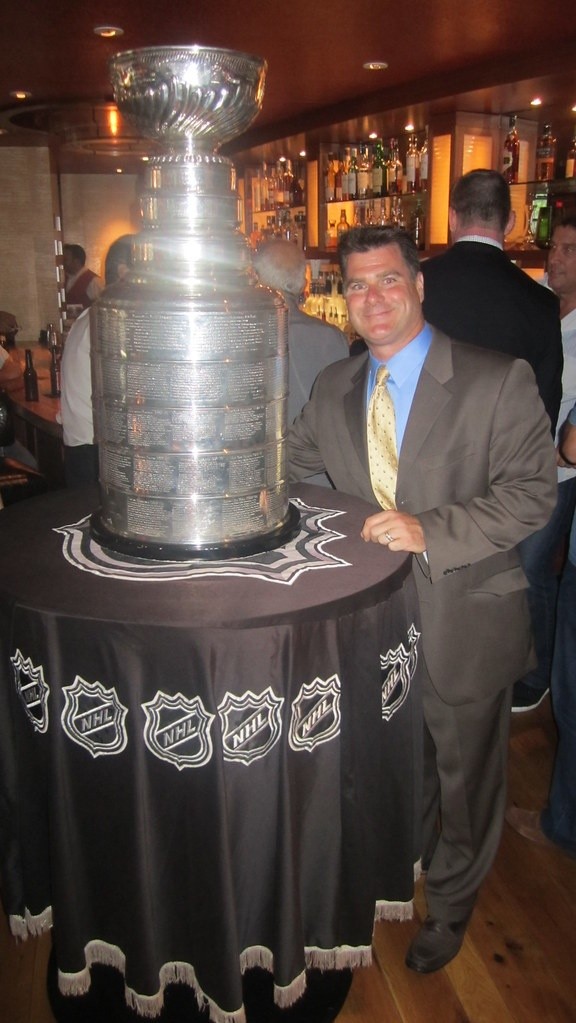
[367,364,399,510]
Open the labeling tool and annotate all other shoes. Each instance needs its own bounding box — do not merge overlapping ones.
[505,806,571,850]
[510,680,550,713]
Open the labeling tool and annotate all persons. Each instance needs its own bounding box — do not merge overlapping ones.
[351,169,576,847]
[284,223,559,975]
[0,234,352,490]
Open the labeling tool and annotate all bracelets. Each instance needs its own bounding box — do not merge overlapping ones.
[558,424,576,465]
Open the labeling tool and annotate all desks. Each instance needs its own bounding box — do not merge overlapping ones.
[0,340,75,461]
[0,481,419,1023]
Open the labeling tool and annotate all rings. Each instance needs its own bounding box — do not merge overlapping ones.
[385,532,395,541]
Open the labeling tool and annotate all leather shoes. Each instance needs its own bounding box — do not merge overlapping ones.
[405,907,470,974]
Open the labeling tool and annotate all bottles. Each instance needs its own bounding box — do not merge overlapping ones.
[324,196,425,252]
[23,349,39,401]
[45,323,60,353]
[297,269,364,346]
[249,156,306,253]
[564,133,576,178]
[324,133,429,201]
[535,120,558,180]
[49,346,61,395]
[502,114,520,185]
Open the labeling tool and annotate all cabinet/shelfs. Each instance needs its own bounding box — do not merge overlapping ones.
[215,67,576,342]
